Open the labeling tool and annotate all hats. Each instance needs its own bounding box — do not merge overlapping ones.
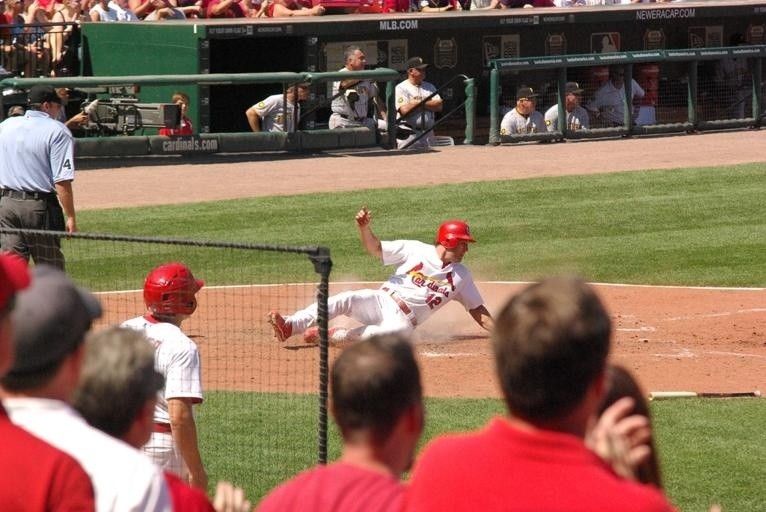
[404,56,430,70]
[566,82,584,93]
[28,86,66,105]
[517,87,539,98]
[0,252,101,373]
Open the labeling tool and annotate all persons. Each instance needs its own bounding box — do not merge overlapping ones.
[268,207,496,350]
[0,84,78,273]
[599,366,661,489]
[402,277,681,511]
[248,333,425,511]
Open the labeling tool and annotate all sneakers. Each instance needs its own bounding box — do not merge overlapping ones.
[305,327,342,343]
[268,313,292,342]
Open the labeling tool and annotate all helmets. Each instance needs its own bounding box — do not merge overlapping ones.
[143,264,204,317]
[436,222,475,249]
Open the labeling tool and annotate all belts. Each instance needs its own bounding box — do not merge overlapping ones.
[384,288,416,326]
[399,122,421,131]
[341,114,364,122]
[150,424,171,433]
[0,189,54,200]
